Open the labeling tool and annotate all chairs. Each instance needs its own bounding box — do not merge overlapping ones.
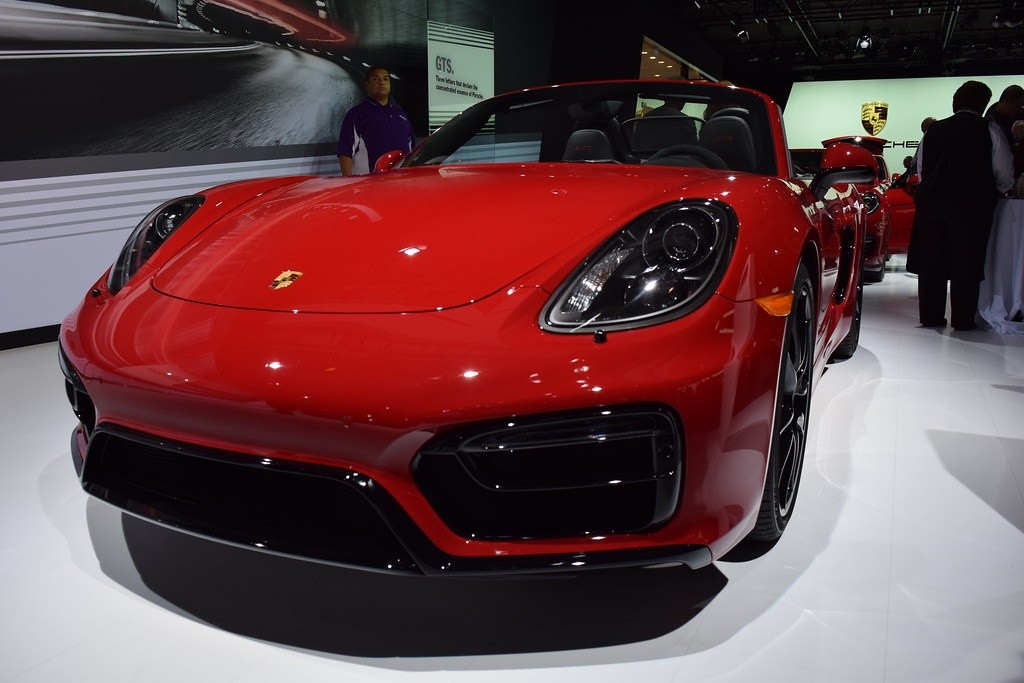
[562,130,615,161]
[697,119,757,173]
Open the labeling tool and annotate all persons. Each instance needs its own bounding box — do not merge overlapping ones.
[903,81,1024,338]
[335,66,415,177]
[645,71,691,119]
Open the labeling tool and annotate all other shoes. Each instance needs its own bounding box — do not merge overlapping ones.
[920,317,948,327]
[953,321,980,331]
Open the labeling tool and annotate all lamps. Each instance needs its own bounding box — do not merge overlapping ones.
[855,33,873,49]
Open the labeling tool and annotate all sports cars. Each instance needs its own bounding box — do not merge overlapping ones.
[190,0,357,58]
[59,76,872,576]
[780,135,924,282]
